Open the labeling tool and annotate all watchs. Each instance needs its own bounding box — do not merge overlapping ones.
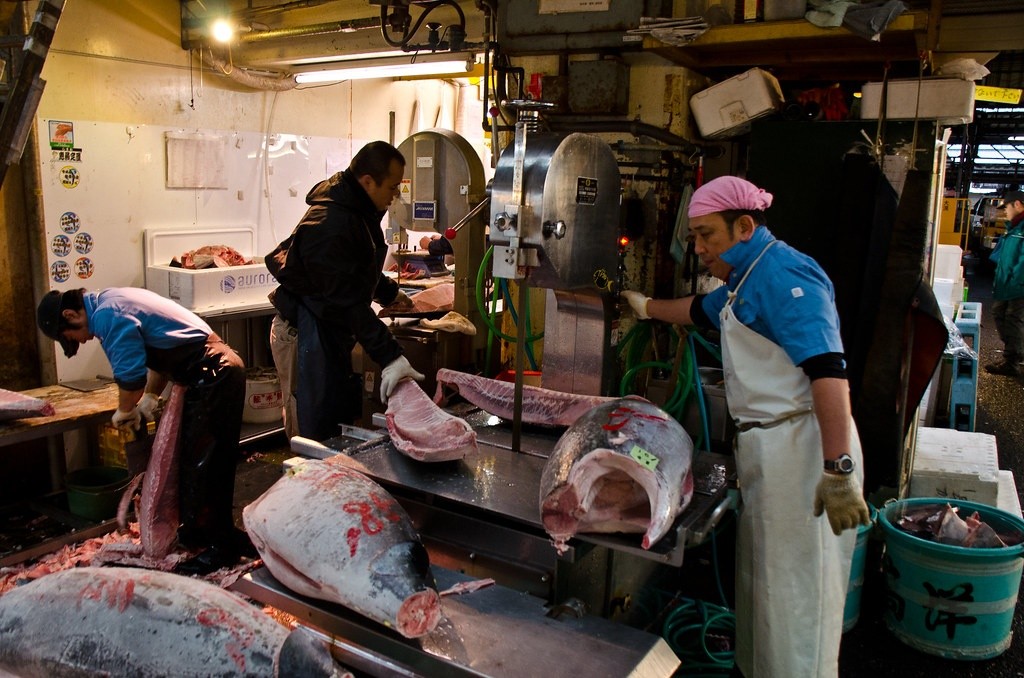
[824,454,855,473]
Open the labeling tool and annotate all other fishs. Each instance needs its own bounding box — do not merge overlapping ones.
[0,388,54,423]
[243,459,443,640]
[114,379,186,561]
[898,501,1007,550]
[539,397,694,555]
[384,379,481,463]
[373,281,456,318]
[434,368,623,429]
[0,564,352,678]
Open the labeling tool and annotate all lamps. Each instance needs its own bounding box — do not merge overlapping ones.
[291,53,478,83]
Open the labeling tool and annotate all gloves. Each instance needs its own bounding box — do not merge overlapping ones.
[616,290,652,319]
[379,355,425,404]
[814,471,869,535]
[137,392,159,422]
[112,408,143,435]
[380,290,412,308]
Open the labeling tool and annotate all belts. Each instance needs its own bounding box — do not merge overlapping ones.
[278,311,298,329]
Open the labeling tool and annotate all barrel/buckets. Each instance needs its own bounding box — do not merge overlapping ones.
[879,496,1024,658]
[244,366,283,424]
[65,465,134,519]
[841,502,879,631]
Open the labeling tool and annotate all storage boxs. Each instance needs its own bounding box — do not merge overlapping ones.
[988,471,1022,521]
[910,426,999,508]
[146,255,280,313]
[931,244,965,323]
[861,78,975,123]
[98,421,156,469]
[690,66,778,140]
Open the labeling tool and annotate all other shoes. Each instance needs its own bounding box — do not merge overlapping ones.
[986,352,1024,377]
[175,547,241,575]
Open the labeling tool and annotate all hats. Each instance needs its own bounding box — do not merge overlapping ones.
[996,190,1024,209]
[36,291,80,358]
[687,175,774,218]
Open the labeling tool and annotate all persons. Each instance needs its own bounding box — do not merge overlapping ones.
[985,191,1024,375]
[268,286,299,440]
[620,176,870,678]
[264,140,425,440]
[36,288,246,575]
[420,235,453,256]
